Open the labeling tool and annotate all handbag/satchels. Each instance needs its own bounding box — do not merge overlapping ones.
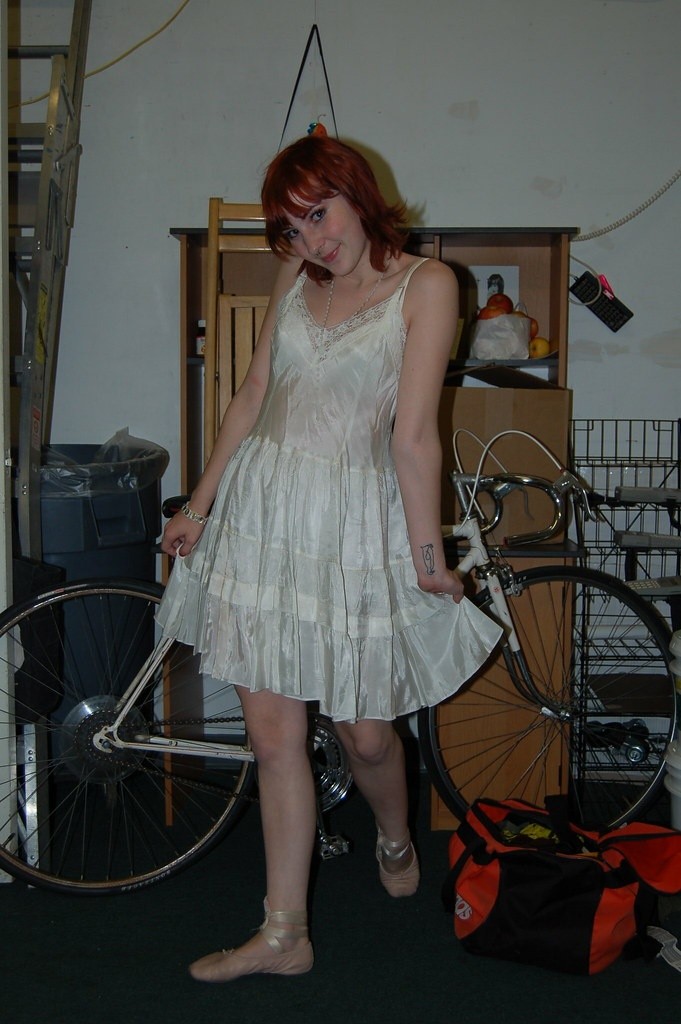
[439,797,681,977]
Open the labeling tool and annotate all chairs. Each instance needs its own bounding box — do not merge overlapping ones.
[203,197,288,472]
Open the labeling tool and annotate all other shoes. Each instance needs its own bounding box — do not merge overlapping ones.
[374,820,420,897]
[188,896,314,982]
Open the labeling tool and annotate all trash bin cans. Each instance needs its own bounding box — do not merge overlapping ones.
[35,442,171,788]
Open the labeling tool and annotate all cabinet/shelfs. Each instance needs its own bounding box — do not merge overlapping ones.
[147,227,585,833]
[569,419,681,828]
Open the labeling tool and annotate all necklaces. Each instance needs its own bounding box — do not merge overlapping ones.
[315,272,381,362]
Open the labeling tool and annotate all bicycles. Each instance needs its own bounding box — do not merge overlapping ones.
[0,429,681,895]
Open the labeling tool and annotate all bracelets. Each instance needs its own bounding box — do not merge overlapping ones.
[182,502,208,525]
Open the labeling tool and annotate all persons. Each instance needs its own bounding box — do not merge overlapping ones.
[486,274,505,298]
[154,135,505,983]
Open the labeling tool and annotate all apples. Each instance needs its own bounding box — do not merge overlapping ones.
[480,295,550,357]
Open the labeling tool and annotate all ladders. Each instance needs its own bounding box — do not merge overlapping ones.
[0,0,92,561]
